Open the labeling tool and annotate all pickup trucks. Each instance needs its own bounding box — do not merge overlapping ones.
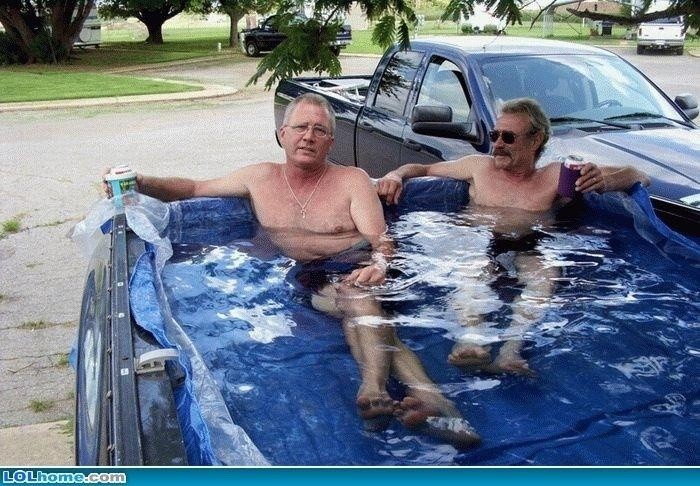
[240,11,353,59]
[272,33,700,229]
[636,8,686,56]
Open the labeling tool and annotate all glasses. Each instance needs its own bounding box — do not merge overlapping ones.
[286,124,333,139]
[488,128,530,144]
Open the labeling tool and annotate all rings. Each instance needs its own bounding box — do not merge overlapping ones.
[592,176,598,183]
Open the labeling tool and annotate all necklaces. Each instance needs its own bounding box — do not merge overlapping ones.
[280,161,329,219]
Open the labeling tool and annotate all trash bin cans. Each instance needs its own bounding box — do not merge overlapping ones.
[602,27,611,35]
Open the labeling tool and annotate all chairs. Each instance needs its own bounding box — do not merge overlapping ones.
[433,62,576,118]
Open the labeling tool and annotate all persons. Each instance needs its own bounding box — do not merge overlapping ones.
[373,97,653,378]
[103,91,481,450]
